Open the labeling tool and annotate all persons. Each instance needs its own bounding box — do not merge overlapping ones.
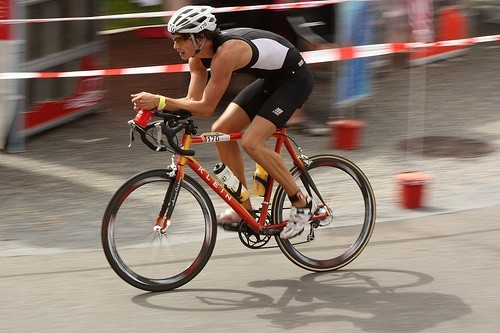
[131,6,318,240]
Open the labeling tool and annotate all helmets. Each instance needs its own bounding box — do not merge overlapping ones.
[168,5,217,33]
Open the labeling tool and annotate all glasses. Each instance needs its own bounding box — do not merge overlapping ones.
[172,37,191,45]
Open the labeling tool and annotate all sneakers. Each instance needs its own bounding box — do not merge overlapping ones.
[279,194,318,239]
[216,207,256,224]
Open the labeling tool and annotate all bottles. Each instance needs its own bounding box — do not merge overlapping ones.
[212,162,250,203]
[251,162,269,197]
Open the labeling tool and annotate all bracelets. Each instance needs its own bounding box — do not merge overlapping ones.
[157,95,166,111]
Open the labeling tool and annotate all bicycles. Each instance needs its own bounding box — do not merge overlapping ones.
[101,109,377,292]
